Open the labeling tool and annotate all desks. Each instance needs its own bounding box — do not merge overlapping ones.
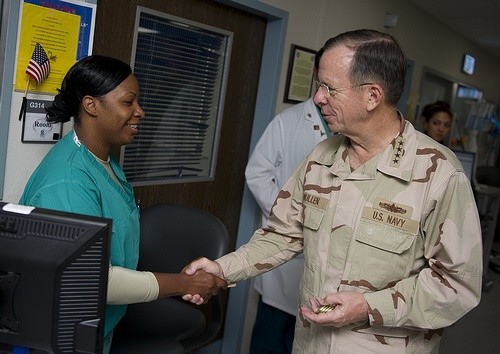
[474,183,500,292]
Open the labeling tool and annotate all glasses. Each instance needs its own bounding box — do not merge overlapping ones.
[315,80,385,96]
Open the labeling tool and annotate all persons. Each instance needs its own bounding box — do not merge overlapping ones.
[245,46,347,354]
[180,28,482,354]
[17,53,228,354]
[424,99,453,141]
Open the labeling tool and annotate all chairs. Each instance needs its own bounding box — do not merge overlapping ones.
[113,203,230,354]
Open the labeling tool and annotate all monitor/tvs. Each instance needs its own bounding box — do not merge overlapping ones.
[0,202,113,354]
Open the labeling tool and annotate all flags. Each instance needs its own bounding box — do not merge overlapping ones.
[24,43,50,85]
